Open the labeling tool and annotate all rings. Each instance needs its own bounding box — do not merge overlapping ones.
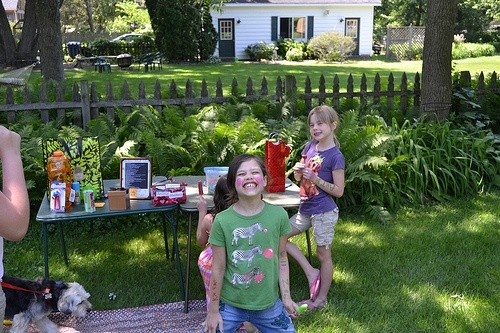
[306,170,308,172]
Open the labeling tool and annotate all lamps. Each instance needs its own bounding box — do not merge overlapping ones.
[340,19,344,22]
[237,19,241,24]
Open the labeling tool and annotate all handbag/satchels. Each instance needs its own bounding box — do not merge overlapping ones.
[265,132,291,193]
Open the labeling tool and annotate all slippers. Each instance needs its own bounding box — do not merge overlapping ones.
[308,271,323,302]
[300,299,318,310]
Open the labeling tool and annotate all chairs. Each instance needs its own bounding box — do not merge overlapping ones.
[93,54,112,73]
[138,51,167,72]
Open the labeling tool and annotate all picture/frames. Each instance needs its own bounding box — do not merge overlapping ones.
[119,156,153,200]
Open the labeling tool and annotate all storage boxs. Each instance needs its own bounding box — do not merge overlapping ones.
[107,187,131,211]
[49,181,66,213]
[204,166,229,194]
[82,189,96,213]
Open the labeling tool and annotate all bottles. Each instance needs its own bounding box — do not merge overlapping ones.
[47,151,73,210]
[71,182,81,206]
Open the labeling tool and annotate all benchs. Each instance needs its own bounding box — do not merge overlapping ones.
[73,56,118,68]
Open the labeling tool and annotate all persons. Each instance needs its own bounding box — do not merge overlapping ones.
[0,125,29,333]
[286,105,345,309]
[196,175,230,313]
[202,154,300,333]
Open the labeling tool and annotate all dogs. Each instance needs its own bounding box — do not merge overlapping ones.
[0,275,91,333]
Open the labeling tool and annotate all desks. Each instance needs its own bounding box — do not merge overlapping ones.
[35,175,314,314]
